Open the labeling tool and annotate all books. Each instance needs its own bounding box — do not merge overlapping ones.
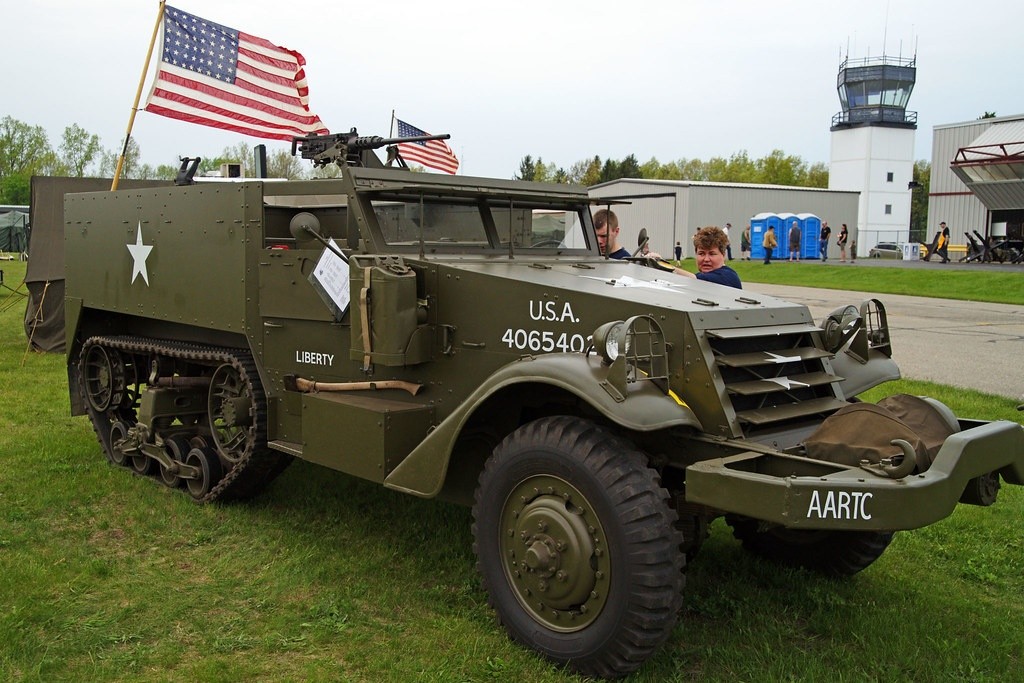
[313,240,350,312]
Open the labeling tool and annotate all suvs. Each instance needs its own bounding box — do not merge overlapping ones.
[870,241,905,260]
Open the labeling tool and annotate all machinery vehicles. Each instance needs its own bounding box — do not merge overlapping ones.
[62,127,1022,682]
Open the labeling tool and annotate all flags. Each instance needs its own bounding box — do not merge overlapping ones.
[396,118,459,176]
[141,2,330,145]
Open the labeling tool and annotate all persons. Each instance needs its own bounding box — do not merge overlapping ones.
[849,240,857,263]
[691,227,701,239]
[658,227,743,289]
[818,221,831,262]
[674,241,683,267]
[722,224,735,261]
[592,209,631,262]
[741,225,752,261]
[641,242,650,258]
[837,224,848,262]
[937,222,951,264]
[763,225,777,265]
[788,221,802,261]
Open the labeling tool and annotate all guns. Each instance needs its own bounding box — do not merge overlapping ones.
[292,127,451,170]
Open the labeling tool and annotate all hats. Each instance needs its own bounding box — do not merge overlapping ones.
[939,222,946,224]
[769,226,774,228]
